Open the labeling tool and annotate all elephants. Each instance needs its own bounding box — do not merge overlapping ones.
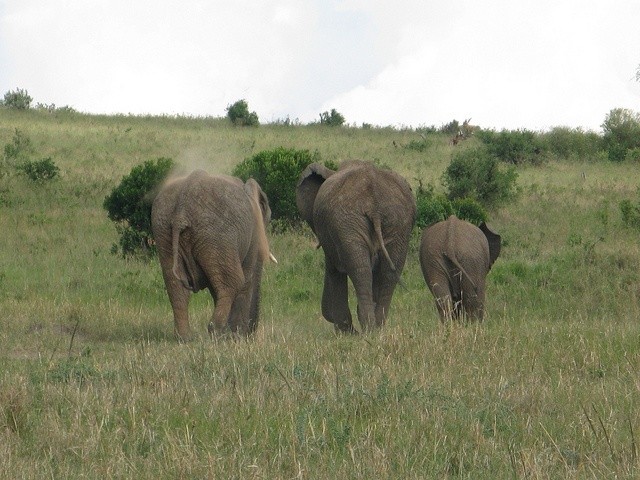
[420,214,503,323]
[296,157,417,336]
[149,168,279,343]
